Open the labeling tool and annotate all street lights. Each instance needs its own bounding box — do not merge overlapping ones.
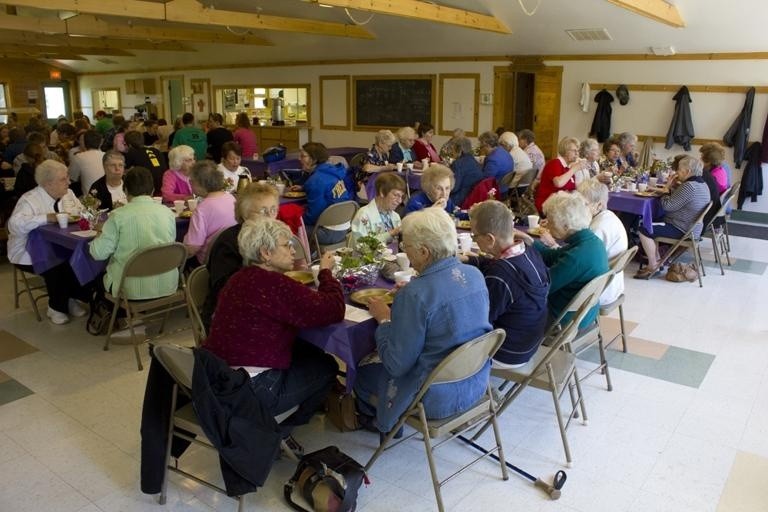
[285,446,370,512]
[324,388,358,432]
[666,262,697,282]
[87,303,119,335]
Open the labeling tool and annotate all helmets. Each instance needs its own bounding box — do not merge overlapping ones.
[526,227,550,236]
[458,221,472,230]
[350,288,395,306]
[282,270,317,284]
[282,191,307,198]
[180,211,192,219]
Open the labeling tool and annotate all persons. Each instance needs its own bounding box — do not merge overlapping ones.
[0,107,357,336]
[459,199,549,375]
[578,179,630,306]
[512,193,609,335]
[350,125,732,279]
[201,217,347,464]
[355,206,492,438]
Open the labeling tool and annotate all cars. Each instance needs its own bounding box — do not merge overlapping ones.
[527,214,539,228]
[173,200,185,213]
[394,271,411,282]
[396,252,410,268]
[605,172,613,184]
[396,163,404,173]
[56,213,69,229]
[188,200,197,211]
[275,182,286,197]
[638,183,649,192]
[153,196,163,203]
[650,177,658,187]
[406,163,414,172]
[421,159,428,169]
[460,233,473,253]
[629,183,637,192]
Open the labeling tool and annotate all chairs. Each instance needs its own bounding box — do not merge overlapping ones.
[365,327,508,510]
[153,344,299,509]
[489,293,587,467]
[68,298,86,317]
[47,304,70,325]
[0,138,744,372]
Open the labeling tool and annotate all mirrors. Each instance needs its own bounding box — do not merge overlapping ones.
[212,84,312,127]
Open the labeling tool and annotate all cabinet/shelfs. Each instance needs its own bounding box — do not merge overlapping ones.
[224,126,260,156]
[125,77,156,95]
[260,126,308,154]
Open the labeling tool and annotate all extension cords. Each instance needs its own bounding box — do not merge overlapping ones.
[237,175,250,193]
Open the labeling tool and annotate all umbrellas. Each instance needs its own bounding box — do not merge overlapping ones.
[640,263,664,271]
[358,414,404,439]
[633,264,660,279]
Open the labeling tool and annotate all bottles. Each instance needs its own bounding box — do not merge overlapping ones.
[616,85,629,105]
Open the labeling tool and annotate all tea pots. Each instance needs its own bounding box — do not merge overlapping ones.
[212,84,312,127]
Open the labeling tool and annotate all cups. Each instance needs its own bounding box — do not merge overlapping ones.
[391,191,405,200]
[399,242,413,250]
[251,205,277,218]
[276,243,291,250]
[470,232,495,241]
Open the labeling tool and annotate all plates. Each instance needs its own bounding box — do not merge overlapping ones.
[125,77,156,95]
[260,126,308,154]
[224,126,260,156]
